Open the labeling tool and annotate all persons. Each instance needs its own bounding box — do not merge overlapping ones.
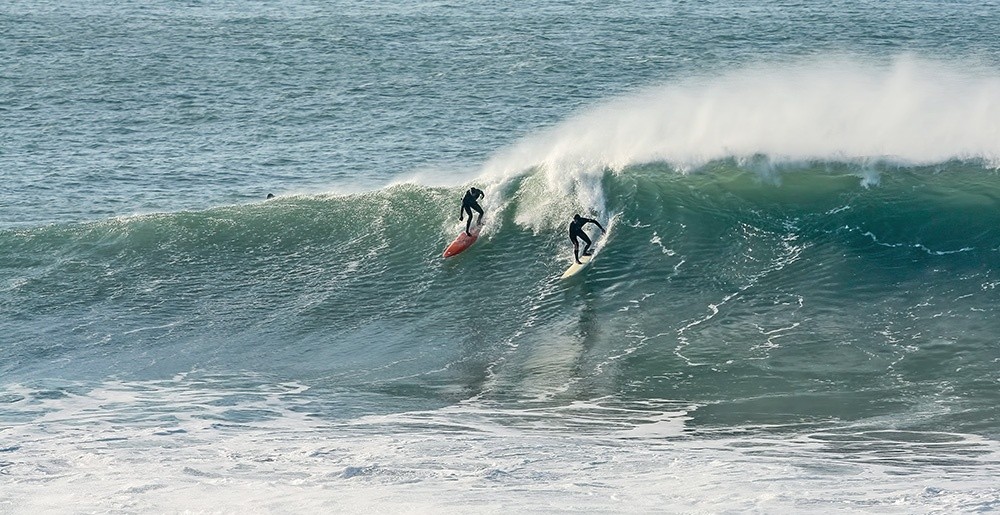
[459,187,484,237]
[569,214,605,264]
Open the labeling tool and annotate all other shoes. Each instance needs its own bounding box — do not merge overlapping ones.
[466,231,472,236]
[584,253,591,256]
[576,260,581,264]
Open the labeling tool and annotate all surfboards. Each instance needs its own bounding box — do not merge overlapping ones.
[560,248,595,279]
[442,220,485,258]
